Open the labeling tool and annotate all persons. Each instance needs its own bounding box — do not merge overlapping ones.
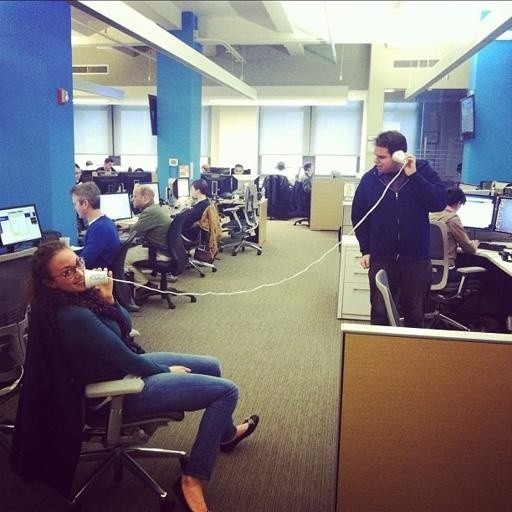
[297,162,316,194]
[68,158,247,303]
[350,129,448,329]
[28,239,260,512]
[272,162,296,188]
[426,185,491,330]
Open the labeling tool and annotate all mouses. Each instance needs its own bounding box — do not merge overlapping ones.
[117,225,122,231]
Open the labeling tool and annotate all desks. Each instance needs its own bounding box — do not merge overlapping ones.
[455,239,512,277]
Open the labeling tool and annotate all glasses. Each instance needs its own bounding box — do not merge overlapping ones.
[51,257,85,280]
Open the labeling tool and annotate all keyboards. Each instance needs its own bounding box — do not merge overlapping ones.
[478,243,507,251]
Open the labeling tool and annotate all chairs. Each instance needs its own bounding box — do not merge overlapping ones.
[375,219,487,331]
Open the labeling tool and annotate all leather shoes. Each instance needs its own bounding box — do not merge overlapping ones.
[173,475,212,512]
[220,415,259,452]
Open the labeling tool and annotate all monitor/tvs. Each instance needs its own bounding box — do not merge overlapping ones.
[456,194,496,230]
[493,196,512,234]
[231,174,260,202]
[459,93,476,142]
[139,182,160,205]
[97,192,133,222]
[93,176,120,195]
[0,203,44,253]
[118,172,152,194]
[201,173,232,203]
[172,177,190,200]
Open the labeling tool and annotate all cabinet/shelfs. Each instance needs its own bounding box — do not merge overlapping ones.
[336,235,373,322]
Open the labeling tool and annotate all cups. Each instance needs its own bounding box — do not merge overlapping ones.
[85,270,108,288]
[391,150,408,164]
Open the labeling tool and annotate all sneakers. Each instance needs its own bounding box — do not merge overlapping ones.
[135,281,157,301]
